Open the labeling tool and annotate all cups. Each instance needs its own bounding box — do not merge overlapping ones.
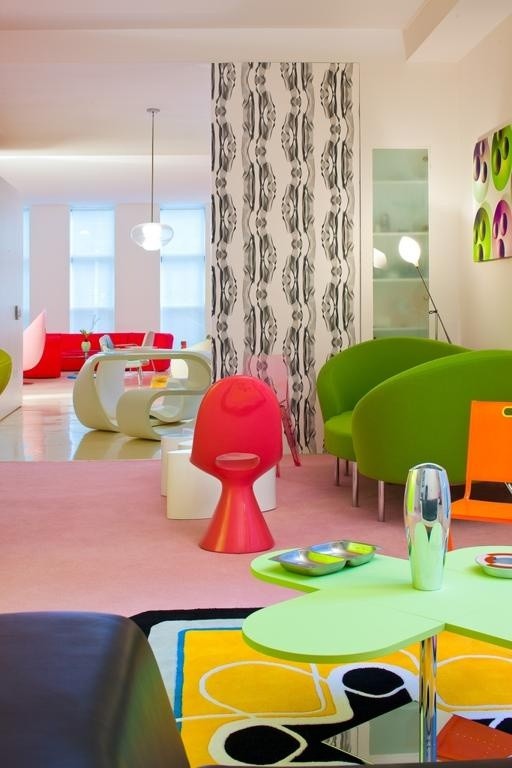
[403,460,453,592]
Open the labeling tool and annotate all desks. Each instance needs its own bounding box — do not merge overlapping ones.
[240,546,512,764]
[161,434,278,520]
[61,350,143,387]
[72,349,213,440]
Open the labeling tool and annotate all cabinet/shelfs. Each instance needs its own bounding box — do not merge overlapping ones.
[372,147,429,340]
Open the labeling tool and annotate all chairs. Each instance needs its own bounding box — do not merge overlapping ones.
[315,337,474,487]
[127,331,156,374]
[190,373,283,553]
[99,334,114,352]
[449,400,512,552]
[349,348,512,522]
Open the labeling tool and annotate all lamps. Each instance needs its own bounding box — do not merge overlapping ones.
[130,106,174,251]
[398,236,452,344]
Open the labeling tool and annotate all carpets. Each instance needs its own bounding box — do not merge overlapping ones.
[129,607,512,766]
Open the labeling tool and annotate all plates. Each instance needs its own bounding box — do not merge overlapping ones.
[475,553,511,580]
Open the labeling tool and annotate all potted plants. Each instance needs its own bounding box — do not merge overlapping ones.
[81,329,96,353]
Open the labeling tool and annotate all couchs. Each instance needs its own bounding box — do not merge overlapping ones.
[24,332,173,379]
